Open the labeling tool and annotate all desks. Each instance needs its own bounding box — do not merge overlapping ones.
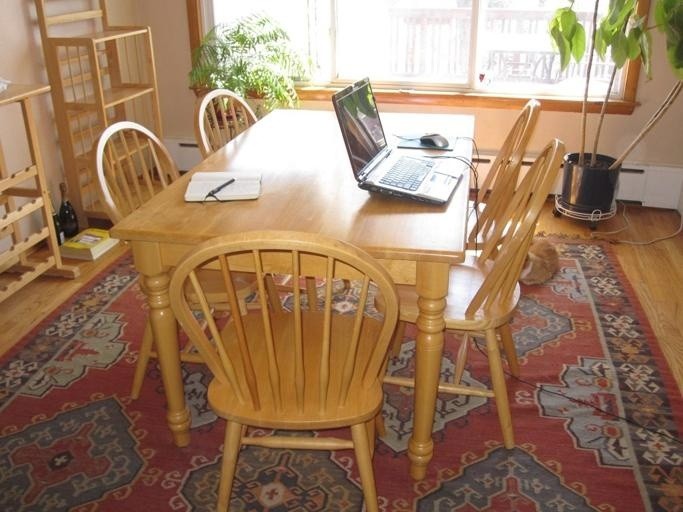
[108,110,476,480]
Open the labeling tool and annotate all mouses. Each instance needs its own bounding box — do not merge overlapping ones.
[419,134,449,148]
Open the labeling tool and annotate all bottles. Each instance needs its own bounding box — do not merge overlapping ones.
[46,182,78,245]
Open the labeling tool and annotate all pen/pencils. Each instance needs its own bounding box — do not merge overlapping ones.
[205,179,235,199]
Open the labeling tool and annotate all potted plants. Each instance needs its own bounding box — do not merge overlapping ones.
[187,10,306,149]
[552,0,683,231]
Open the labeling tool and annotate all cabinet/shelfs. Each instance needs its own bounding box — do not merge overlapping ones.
[36,0,165,230]
[1,79,80,310]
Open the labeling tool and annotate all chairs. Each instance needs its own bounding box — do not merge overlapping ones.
[95,119,267,400]
[194,89,258,158]
[373,138,565,450]
[466,98,543,261]
[167,230,400,512]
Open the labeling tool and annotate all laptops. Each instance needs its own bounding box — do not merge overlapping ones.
[332,77,467,204]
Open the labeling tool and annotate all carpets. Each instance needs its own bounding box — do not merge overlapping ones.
[0,235,683,512]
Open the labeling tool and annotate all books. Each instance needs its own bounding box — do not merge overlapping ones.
[58,228,120,261]
[183,171,262,203]
